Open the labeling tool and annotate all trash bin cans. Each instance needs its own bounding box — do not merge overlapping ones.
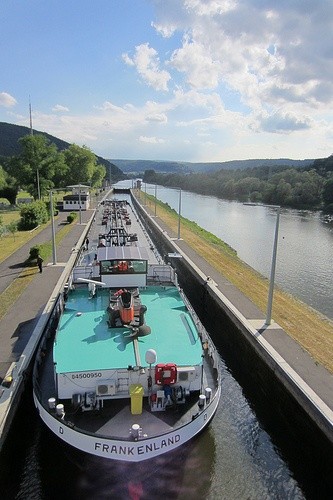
[129,384,144,414]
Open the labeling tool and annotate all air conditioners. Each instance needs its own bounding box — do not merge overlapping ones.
[176,367,196,383]
[95,380,117,396]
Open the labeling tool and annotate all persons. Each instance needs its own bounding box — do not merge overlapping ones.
[37,255,44,273]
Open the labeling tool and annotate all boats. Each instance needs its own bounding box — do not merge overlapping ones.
[32,198,222,464]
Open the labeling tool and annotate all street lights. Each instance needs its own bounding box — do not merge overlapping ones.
[242,202,280,326]
